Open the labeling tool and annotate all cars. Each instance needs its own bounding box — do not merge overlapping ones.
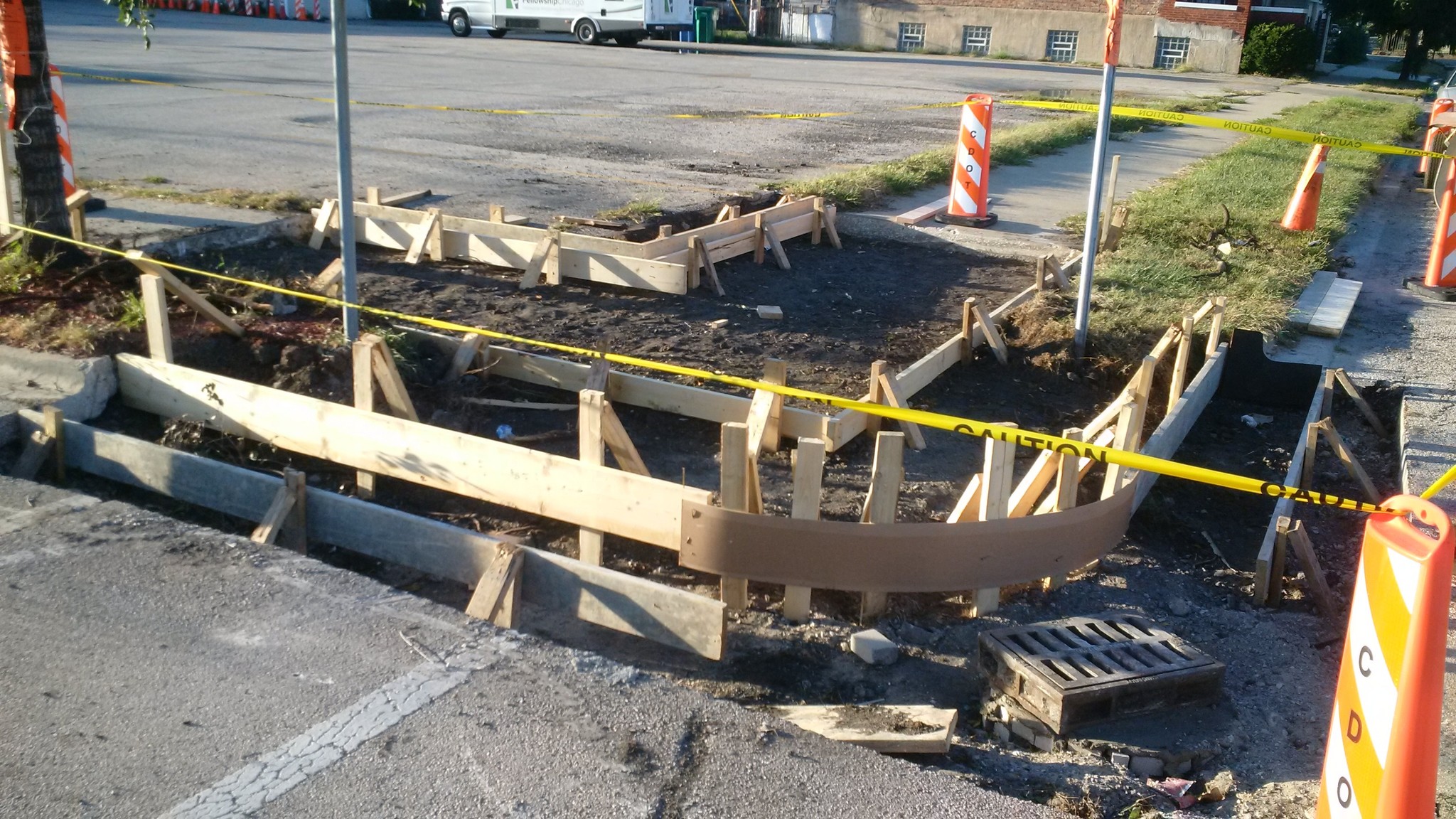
[1430,66,1456,103]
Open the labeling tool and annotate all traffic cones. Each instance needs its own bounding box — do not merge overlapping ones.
[1278,131,1331,233]
[297,3,308,21]
[292,0,302,20]
[312,0,323,22]
[147,0,262,17]
[267,0,277,19]
[278,0,288,20]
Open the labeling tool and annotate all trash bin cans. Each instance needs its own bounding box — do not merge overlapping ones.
[694,6,719,43]
[679,5,696,41]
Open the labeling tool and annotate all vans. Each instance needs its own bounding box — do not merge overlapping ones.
[441,0,694,48]
